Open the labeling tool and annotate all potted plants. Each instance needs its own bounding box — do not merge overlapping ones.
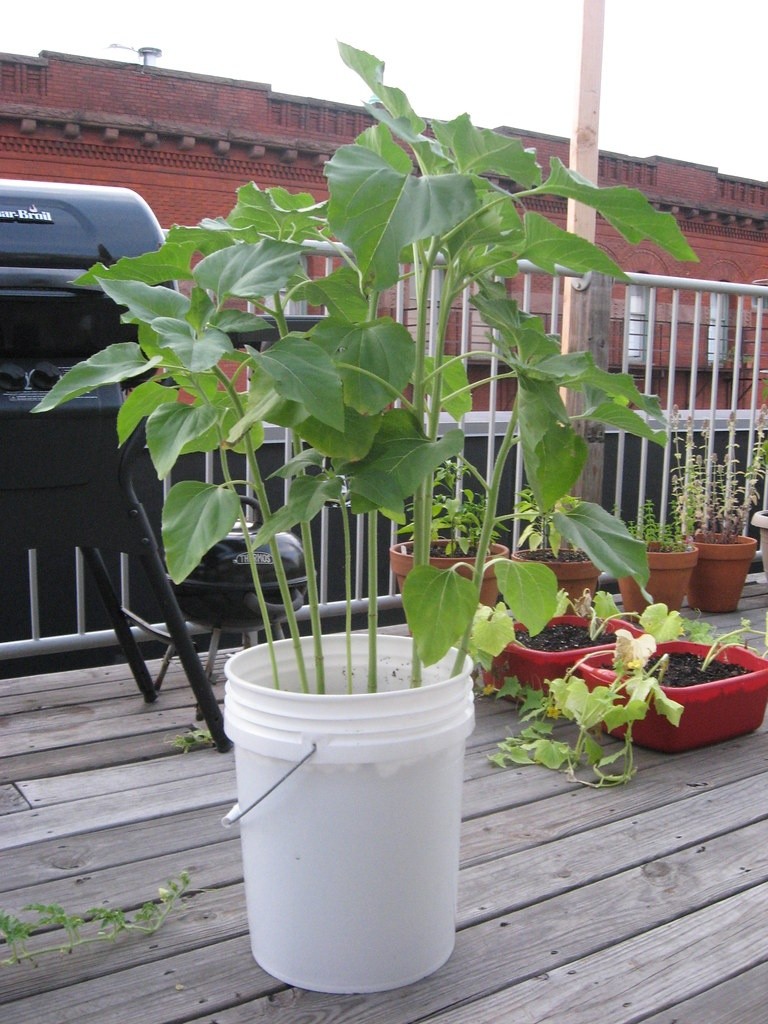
[41,40,768,993]
